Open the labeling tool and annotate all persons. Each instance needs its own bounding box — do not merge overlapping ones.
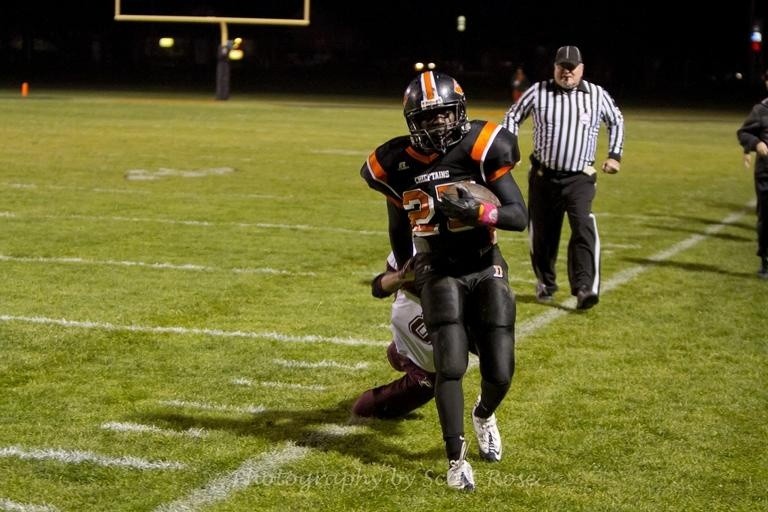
[736,97,768,273]
[502,44,625,309]
[212,39,235,100]
[510,66,528,106]
[360,69,530,491]
[743,145,761,259]
[348,238,437,417]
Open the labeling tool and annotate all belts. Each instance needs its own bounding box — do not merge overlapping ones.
[529,153,583,180]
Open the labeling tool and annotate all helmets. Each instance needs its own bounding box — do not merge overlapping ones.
[403,70,472,136]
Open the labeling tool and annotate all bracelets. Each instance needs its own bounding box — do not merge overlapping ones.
[477,201,499,227]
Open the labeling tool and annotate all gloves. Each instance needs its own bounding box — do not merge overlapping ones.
[435,186,481,225]
[399,252,441,285]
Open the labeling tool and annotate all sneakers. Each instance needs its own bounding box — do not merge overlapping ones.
[471,395,503,463]
[537,285,554,300]
[447,460,476,491]
[576,287,599,309]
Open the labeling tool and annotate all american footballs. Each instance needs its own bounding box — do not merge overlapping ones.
[443,181,502,210]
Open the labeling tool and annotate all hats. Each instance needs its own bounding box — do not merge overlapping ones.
[555,45,583,69]
